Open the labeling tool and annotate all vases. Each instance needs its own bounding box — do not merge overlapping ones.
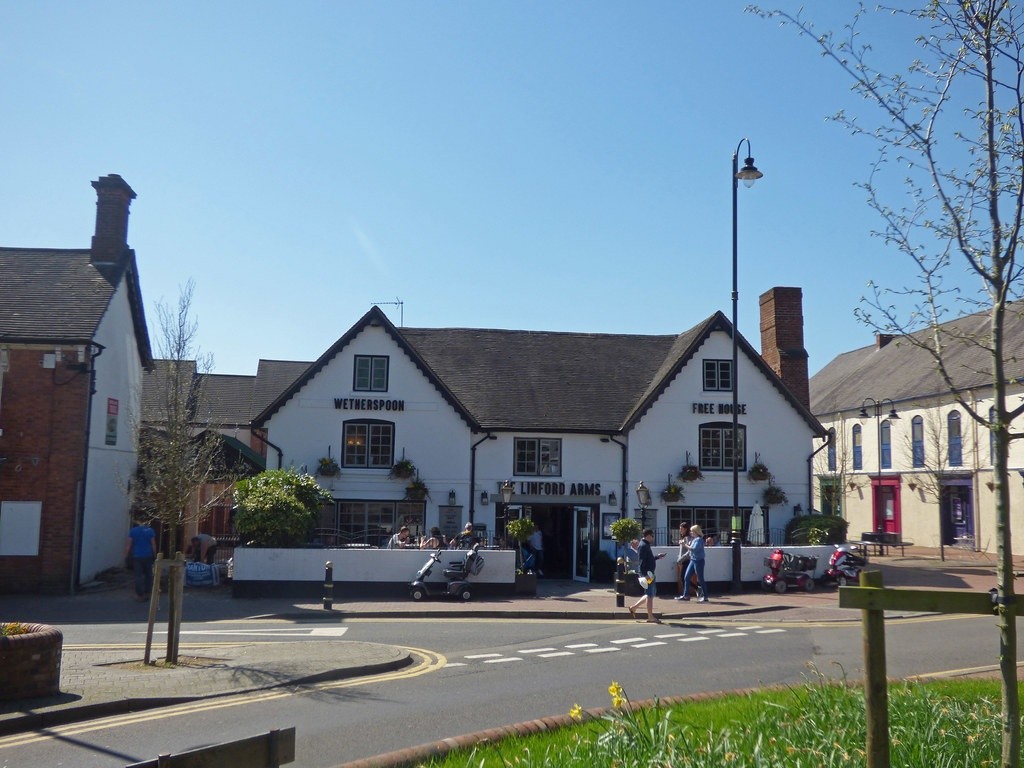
[410,489,425,500]
[768,498,782,504]
[753,476,768,480]
[686,476,697,480]
[665,497,679,502]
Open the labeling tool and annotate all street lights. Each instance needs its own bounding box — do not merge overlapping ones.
[732,138,764,596]
[859,398,901,556]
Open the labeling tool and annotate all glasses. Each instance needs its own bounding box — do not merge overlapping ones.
[649,534,654,538]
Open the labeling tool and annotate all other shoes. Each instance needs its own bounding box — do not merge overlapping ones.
[674,595,684,600]
[138,593,148,601]
[697,600,708,604]
[696,586,702,598]
[538,569,544,576]
[678,597,690,602]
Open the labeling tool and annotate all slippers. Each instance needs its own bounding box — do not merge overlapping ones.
[629,607,636,619]
[647,619,661,624]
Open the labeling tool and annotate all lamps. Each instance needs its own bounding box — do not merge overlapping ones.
[449,490,455,505]
[609,490,617,506]
[481,491,488,506]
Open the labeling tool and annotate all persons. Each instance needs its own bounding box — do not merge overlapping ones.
[420,523,510,550]
[675,522,709,602]
[532,525,544,575]
[706,534,720,545]
[629,530,665,623]
[191,534,217,564]
[387,528,410,549]
[127,515,157,600]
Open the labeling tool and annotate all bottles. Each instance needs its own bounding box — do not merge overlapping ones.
[647,576,654,584]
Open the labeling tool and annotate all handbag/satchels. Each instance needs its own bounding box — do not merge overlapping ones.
[638,571,654,590]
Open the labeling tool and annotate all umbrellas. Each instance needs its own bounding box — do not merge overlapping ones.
[747,501,764,545]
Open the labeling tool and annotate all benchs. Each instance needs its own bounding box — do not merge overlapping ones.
[851,532,914,556]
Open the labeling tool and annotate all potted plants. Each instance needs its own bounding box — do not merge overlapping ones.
[505,516,537,595]
[609,518,641,596]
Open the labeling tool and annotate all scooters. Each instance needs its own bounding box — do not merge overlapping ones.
[821,543,862,587]
[408,543,486,600]
[760,543,819,593]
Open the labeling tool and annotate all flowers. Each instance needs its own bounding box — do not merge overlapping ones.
[315,457,343,480]
[763,486,788,506]
[660,482,686,504]
[387,458,432,504]
[747,464,775,484]
[678,464,705,483]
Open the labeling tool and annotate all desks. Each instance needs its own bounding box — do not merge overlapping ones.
[346,544,370,548]
[406,544,420,549]
